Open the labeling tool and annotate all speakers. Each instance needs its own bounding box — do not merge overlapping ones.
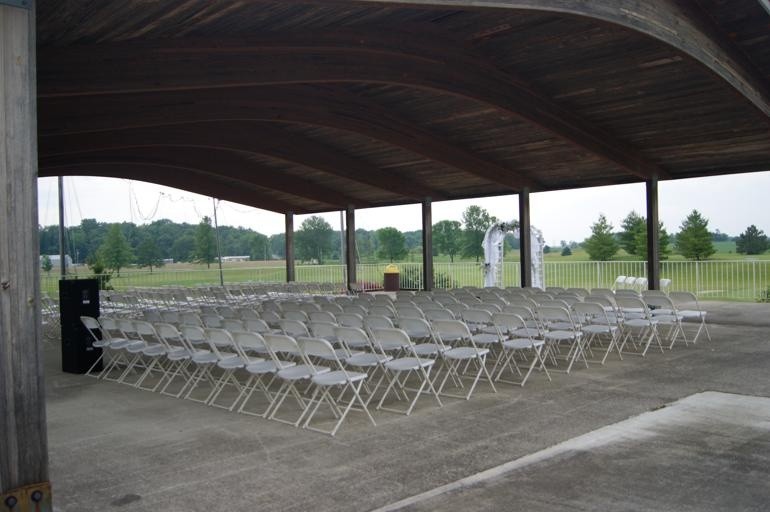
[59,279,103,373]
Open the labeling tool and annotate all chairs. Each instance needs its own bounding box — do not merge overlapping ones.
[660,279,671,290]
[79,282,712,435]
[610,276,649,289]
[41,291,62,342]
